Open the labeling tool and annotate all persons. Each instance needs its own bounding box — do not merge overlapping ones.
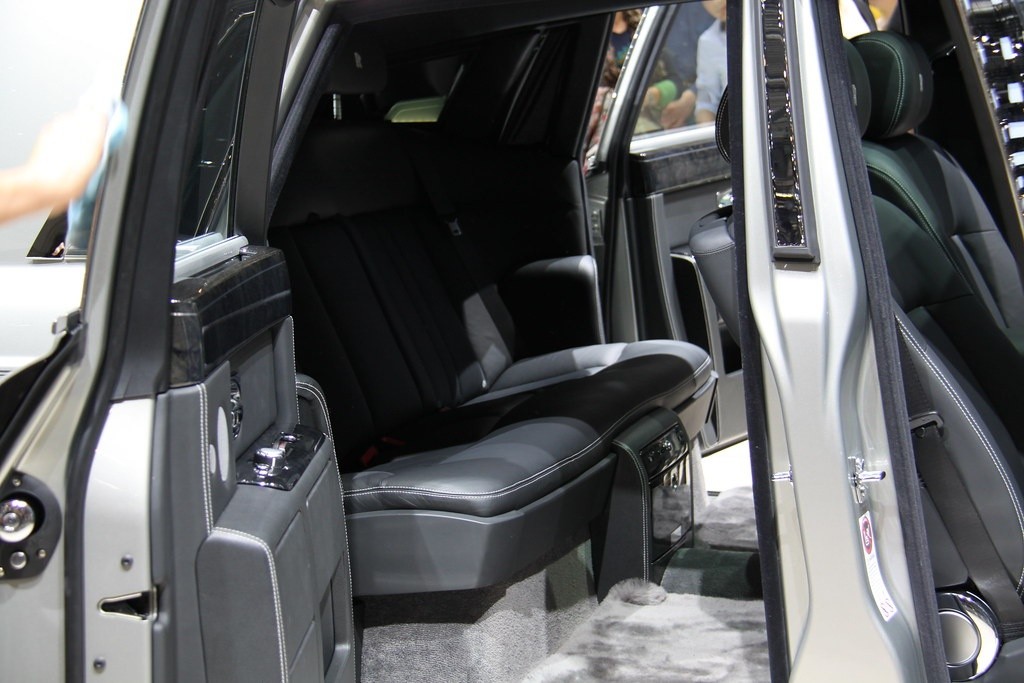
[582,0,903,174]
[0,101,109,224]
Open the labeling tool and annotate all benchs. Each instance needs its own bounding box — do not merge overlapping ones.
[268,203,717,597]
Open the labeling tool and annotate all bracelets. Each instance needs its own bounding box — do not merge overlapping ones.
[645,91,653,105]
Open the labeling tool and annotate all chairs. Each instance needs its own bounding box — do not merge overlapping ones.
[719,33,1024,641]
